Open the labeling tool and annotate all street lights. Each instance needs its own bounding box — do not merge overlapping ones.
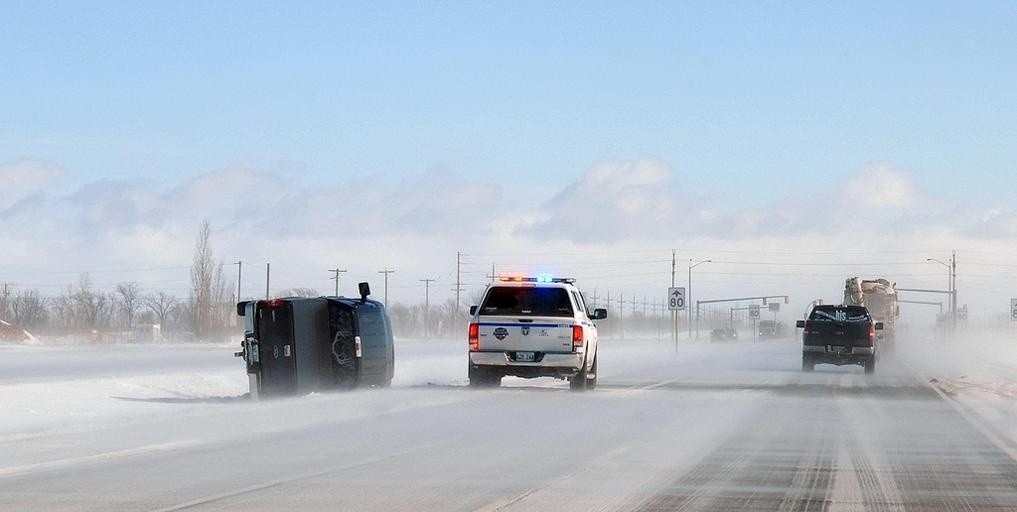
[926,258,951,310]
[687,259,712,336]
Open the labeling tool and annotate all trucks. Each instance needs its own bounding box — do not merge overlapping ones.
[841,277,900,358]
[758,320,794,342]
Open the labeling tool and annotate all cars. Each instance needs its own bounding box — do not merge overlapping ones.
[710,328,727,343]
[237,281,395,401]
[467,275,607,392]
[726,329,737,341]
[795,303,883,375]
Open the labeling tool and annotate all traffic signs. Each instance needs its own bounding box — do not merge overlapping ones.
[667,287,685,310]
